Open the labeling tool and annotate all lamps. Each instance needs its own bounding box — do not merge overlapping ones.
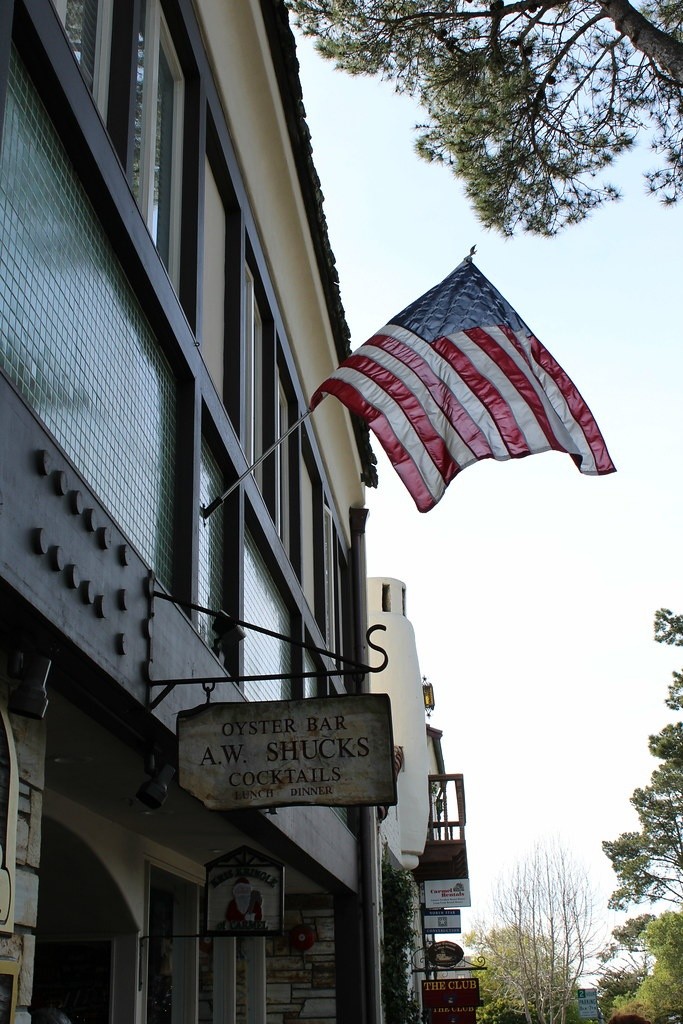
[210,610,246,659]
[135,730,169,809]
[6,647,52,721]
[422,675,435,718]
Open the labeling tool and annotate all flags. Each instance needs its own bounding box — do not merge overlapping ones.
[307,261,619,513]
